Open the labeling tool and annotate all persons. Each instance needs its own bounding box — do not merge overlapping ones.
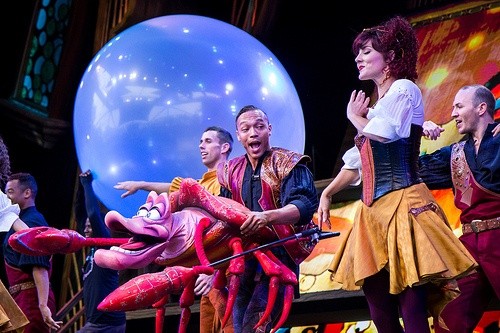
[113,127,235,333]
[4,173,64,333]
[0,140,29,333]
[317,18,479,333]
[419,85,500,333]
[217,104,320,333]
[74,169,126,333]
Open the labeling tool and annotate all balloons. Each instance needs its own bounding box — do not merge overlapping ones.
[72,13,306,218]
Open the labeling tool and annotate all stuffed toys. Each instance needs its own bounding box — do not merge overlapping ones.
[8,175,299,332]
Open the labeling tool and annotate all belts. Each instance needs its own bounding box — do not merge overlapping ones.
[9,281,36,294]
[462,217,500,233]
